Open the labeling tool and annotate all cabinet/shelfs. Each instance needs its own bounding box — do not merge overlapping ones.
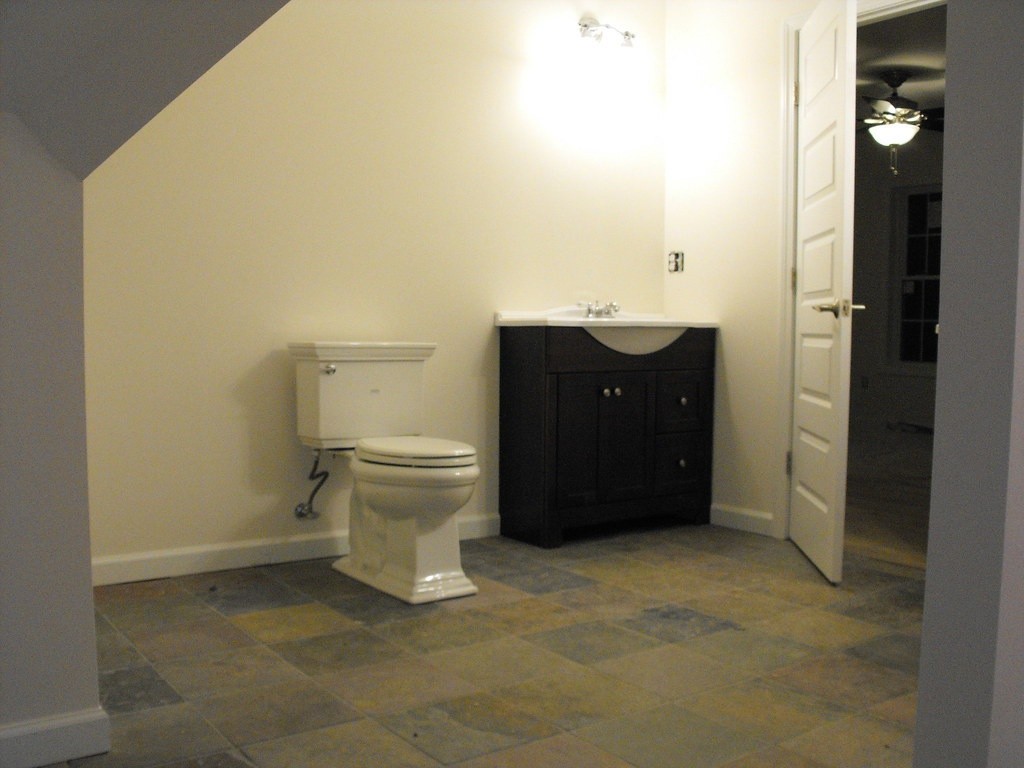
[499,327,717,549]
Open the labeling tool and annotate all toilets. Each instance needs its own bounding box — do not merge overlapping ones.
[287,341,480,605]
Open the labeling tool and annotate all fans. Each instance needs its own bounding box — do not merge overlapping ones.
[855,68,944,130]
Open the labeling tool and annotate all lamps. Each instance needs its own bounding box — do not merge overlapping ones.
[578,16,637,47]
[868,122,920,176]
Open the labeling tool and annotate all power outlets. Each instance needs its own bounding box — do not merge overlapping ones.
[668,251,684,273]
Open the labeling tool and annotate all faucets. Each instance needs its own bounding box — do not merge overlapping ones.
[578,300,619,318]
